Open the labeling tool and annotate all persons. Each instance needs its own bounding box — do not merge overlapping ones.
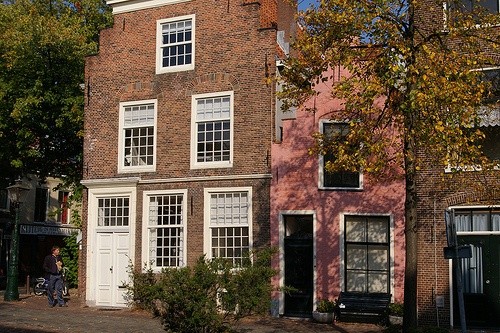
[44,246,69,308]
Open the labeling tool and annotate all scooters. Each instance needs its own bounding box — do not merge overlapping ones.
[34,266,69,296]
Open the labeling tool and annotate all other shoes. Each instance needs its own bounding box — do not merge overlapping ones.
[49,303,69,308]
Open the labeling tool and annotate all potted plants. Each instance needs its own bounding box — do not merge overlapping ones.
[387,302,404,326]
[313,297,335,323]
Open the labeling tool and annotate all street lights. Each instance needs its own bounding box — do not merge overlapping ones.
[3,176,33,302]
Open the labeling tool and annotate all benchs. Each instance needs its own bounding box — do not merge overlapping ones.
[334,290,392,325]
